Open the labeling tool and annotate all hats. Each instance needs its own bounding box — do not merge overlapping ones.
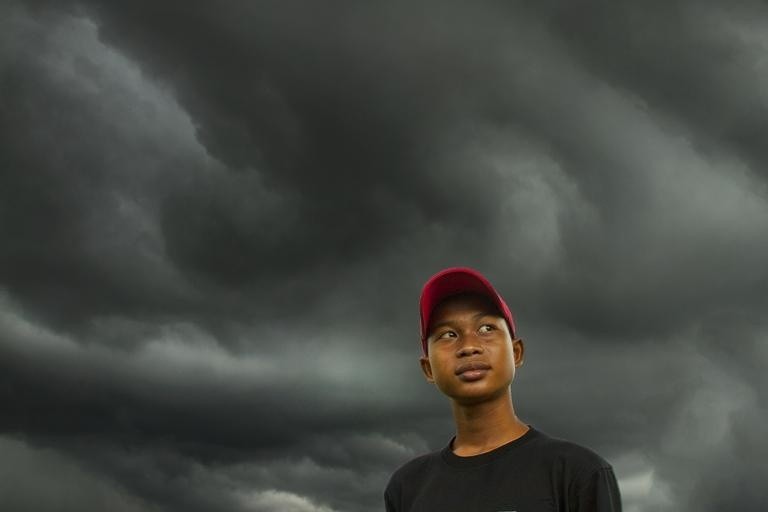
[417,268,512,338]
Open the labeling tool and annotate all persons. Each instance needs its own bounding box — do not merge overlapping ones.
[384,266,623,511]
